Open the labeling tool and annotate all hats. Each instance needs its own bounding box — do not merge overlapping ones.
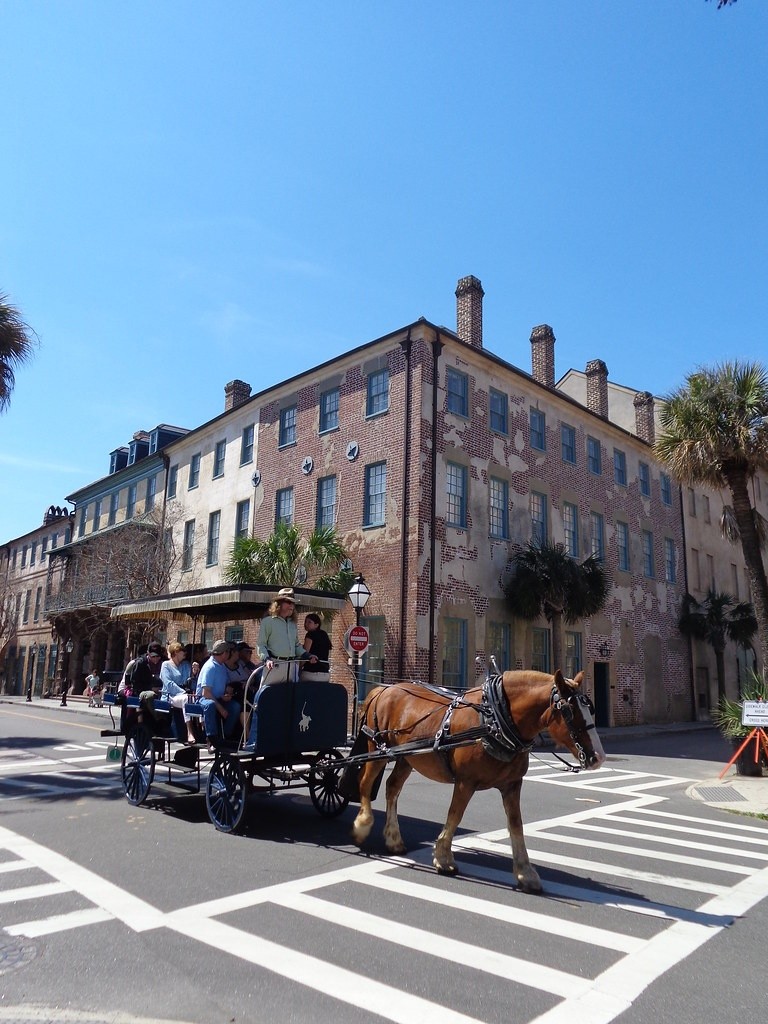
[212,640,234,654]
[238,642,255,650]
[272,588,300,603]
[148,642,162,657]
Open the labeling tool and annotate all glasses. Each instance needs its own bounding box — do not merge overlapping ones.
[152,656,159,658]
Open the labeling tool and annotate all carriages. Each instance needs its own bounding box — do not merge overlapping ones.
[100,584,607,894]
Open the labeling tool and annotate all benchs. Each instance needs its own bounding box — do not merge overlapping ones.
[102,680,225,739]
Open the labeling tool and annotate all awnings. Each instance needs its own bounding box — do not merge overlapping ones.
[110,584,346,664]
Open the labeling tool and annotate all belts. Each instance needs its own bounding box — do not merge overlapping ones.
[271,655,295,661]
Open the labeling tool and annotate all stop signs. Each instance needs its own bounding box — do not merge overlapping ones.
[347,626,370,654]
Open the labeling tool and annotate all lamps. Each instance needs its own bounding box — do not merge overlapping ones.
[599,641,612,659]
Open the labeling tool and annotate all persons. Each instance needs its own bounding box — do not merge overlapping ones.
[84,669,103,708]
[300,614,334,682]
[117,639,264,749]
[256,588,320,689]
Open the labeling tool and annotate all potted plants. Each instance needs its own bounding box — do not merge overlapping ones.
[707,664,768,776]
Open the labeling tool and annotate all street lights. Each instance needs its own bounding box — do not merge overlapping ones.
[349,576,372,742]
[61,638,74,706]
[26,642,39,702]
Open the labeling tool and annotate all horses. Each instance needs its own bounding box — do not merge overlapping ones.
[353,668,608,892]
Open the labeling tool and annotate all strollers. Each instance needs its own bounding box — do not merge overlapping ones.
[88,686,103,708]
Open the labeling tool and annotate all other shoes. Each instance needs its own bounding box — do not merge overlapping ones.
[188,736,195,743]
[208,745,216,753]
[242,741,256,750]
